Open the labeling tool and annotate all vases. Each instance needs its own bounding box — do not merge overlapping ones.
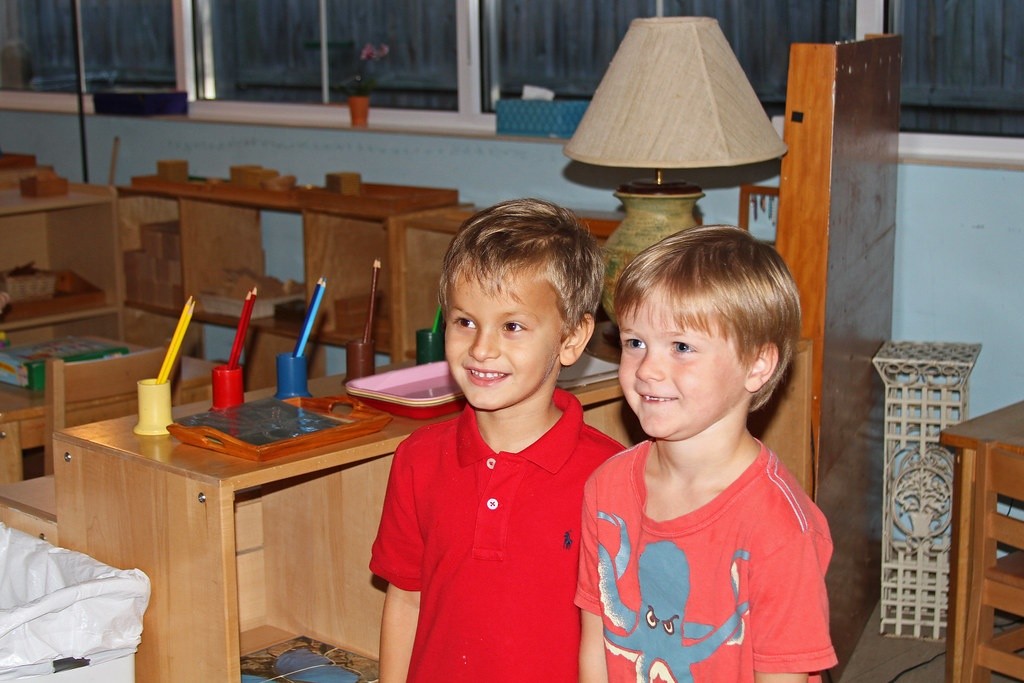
[347,96,372,129]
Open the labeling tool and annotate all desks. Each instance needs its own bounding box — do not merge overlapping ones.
[0,336,234,485]
[940,400,1024,683]
[54,342,665,683]
[384,202,702,364]
[873,339,981,644]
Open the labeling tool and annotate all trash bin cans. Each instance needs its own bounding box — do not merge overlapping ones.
[0,523,151,683]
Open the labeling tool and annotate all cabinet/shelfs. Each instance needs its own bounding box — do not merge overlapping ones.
[113,172,460,365]
[0,165,126,346]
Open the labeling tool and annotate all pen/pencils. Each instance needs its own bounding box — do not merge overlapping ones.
[363,258,381,343]
[227,286,257,370]
[292,276,327,358]
[432,303,444,333]
[156,295,196,384]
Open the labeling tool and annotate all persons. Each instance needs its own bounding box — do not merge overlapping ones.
[571,224,839,683]
[369,197,633,683]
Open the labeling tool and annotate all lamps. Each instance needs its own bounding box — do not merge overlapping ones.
[562,17,789,345]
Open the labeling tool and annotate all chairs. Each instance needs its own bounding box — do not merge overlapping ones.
[960,440,1024,683]
[0,344,183,548]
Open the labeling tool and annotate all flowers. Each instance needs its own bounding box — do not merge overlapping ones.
[356,42,390,96]
